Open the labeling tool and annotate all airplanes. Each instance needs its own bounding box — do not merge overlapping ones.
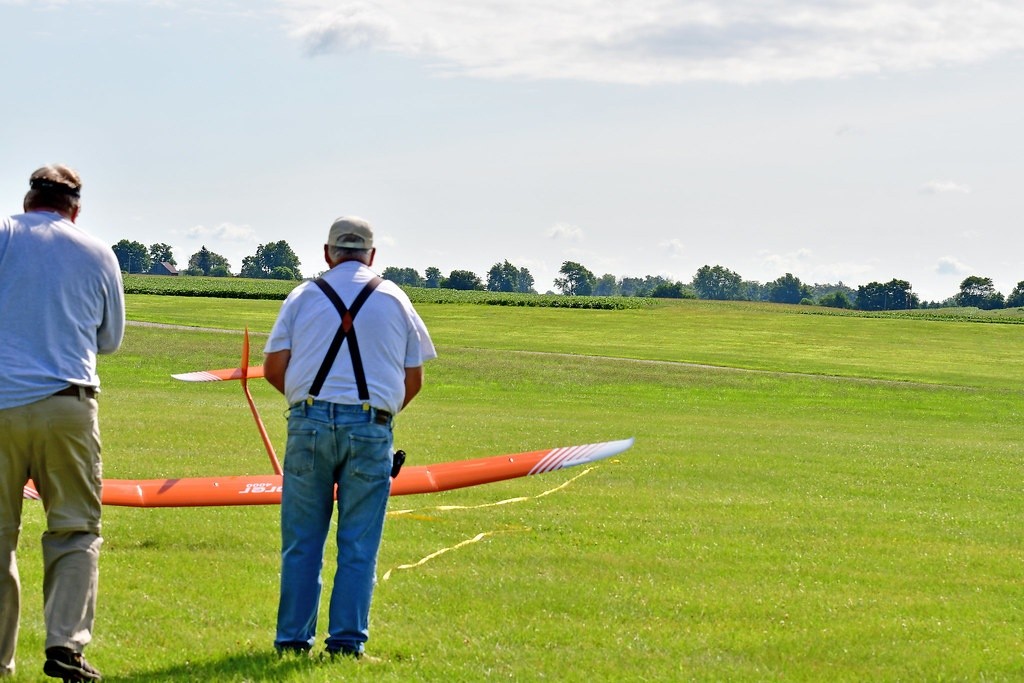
[23,325,632,507]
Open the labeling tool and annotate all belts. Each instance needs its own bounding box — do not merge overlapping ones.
[53,386,94,398]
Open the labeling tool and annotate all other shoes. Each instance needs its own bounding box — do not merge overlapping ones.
[274,644,315,659]
[330,651,383,665]
[44,646,103,683]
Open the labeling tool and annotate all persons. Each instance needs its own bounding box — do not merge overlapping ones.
[0,167,124,683]
[263,215,439,664]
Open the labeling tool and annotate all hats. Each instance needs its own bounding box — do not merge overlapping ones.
[29,164,82,197]
[327,217,374,249]
